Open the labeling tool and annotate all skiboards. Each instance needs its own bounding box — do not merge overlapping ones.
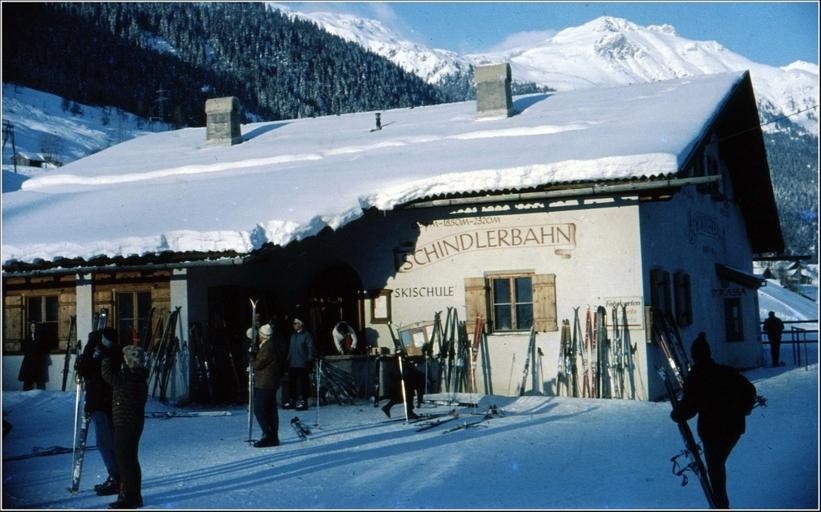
[92,308,107,330]
[291,416,313,440]
[62,316,75,391]
[315,360,323,428]
[247,299,259,444]
[69,375,90,493]
[518,302,635,400]
[656,312,690,393]
[390,309,492,421]
[137,307,180,403]
[652,360,719,508]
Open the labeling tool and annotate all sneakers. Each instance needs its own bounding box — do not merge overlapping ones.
[253,438,280,448]
[381,406,391,418]
[409,412,418,418]
[110,496,143,509]
[95,479,118,495]
[282,399,309,410]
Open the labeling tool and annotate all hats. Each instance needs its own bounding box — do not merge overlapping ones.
[691,332,712,358]
[258,324,272,338]
[122,345,144,368]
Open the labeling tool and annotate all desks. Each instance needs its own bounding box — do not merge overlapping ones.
[324,355,370,399]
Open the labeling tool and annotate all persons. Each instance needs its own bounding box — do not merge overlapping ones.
[763,311,784,367]
[671,331,755,508]
[381,341,431,419]
[108,347,148,509]
[18,322,53,391]
[282,315,314,411]
[245,321,287,447]
[76,326,134,497]
[332,320,357,355]
